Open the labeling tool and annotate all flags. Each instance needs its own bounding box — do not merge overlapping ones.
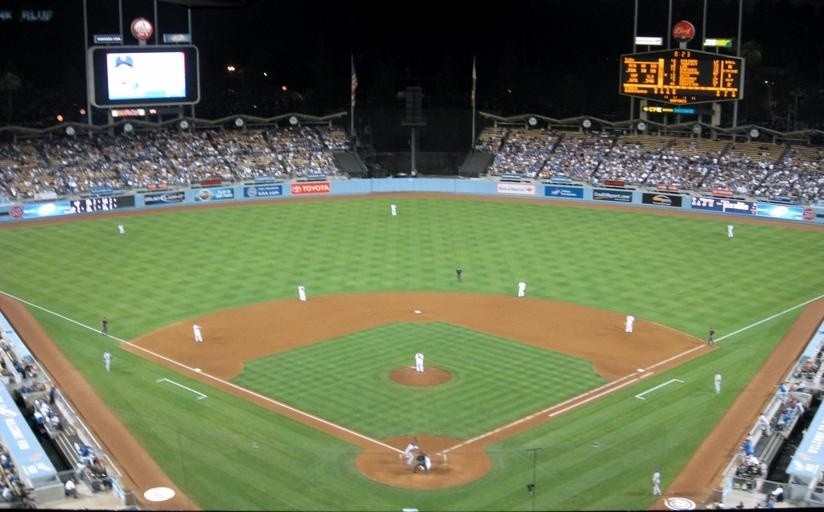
[350,65,360,107]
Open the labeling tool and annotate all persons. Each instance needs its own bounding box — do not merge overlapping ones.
[456,267,463,284]
[0,120,350,206]
[708,323,824,509]
[298,282,307,302]
[475,125,824,206]
[625,313,635,333]
[391,203,398,217]
[652,469,662,496]
[518,280,527,297]
[728,224,734,239]
[118,223,126,234]
[193,322,204,343]
[1,317,113,505]
[398,441,432,474]
[415,351,425,373]
